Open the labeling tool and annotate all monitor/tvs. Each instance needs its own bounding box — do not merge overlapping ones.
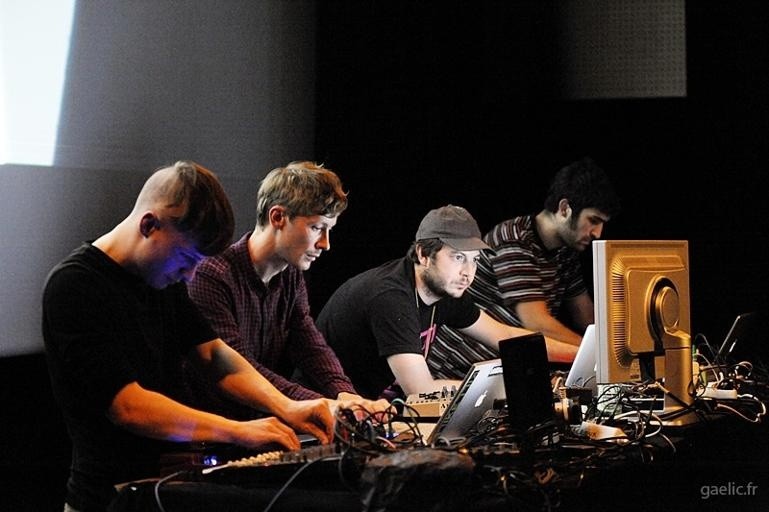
[591,240,699,429]
[498,332,553,434]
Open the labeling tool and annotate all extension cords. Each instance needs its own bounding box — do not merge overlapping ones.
[579,421,629,447]
[694,382,738,402]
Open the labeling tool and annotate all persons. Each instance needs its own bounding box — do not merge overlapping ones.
[186,159,396,425]
[427,160,621,386]
[311,202,582,404]
[41,160,348,512]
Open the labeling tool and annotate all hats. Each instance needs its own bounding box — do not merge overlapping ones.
[415,204,497,256]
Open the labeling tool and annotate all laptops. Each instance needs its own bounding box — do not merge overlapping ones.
[563,324,596,396]
[373,358,506,449]
[709,312,750,366]
